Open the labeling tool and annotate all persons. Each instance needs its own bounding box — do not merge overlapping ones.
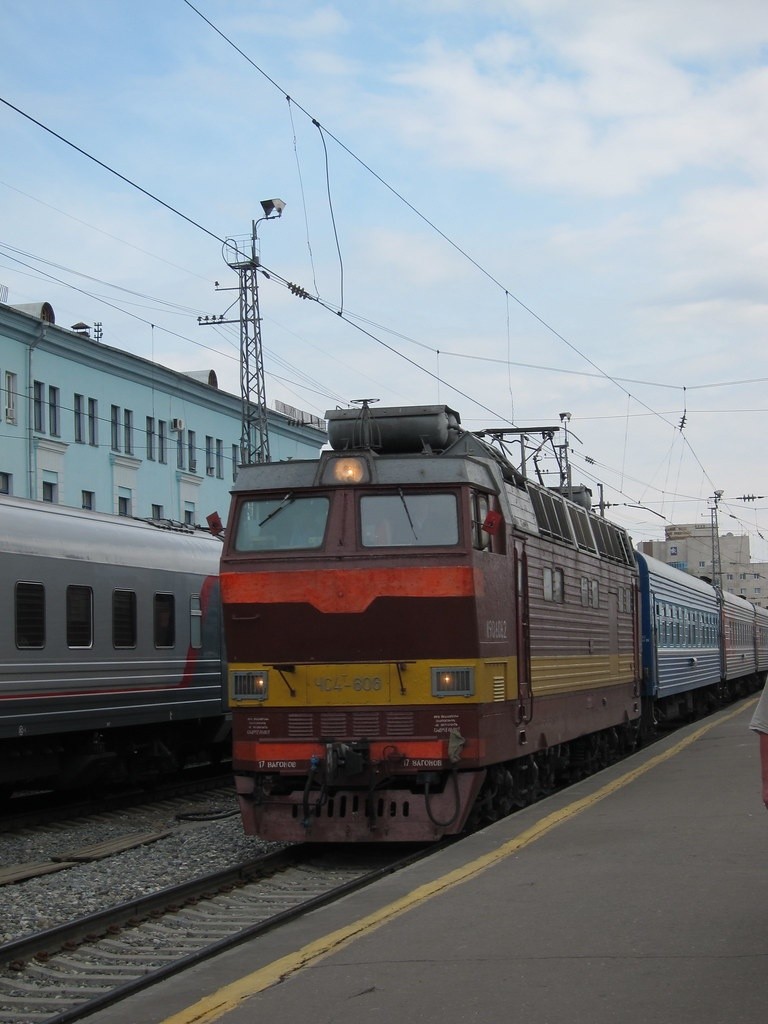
[748,673,768,809]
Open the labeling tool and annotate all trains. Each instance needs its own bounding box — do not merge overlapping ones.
[0,491,229,792]
[208,394,766,850]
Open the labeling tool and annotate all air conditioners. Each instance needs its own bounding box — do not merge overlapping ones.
[172,417,184,431]
